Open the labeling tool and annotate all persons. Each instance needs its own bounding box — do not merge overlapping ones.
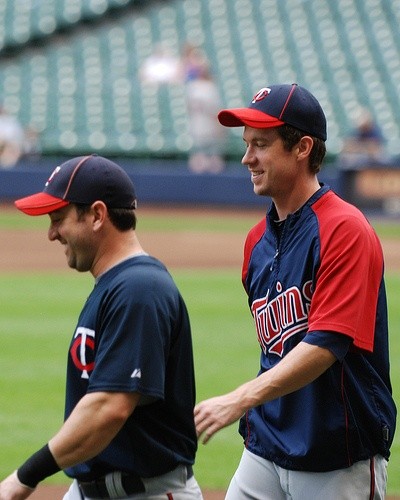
[0,155,203,500]
[194,83,397,500]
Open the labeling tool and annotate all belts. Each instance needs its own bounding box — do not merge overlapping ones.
[77,464,195,500]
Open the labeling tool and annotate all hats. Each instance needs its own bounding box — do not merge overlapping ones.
[217,83,327,141]
[13,152,137,216]
